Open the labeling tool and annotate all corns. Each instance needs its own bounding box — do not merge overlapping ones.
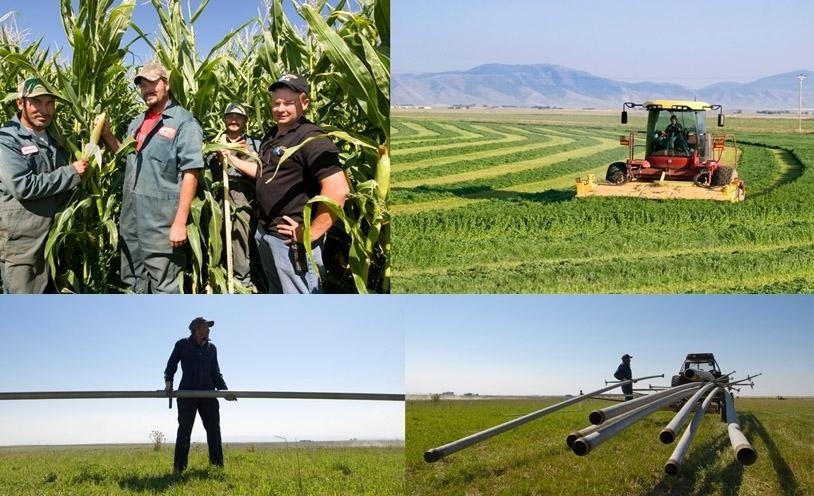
[88,112,106,146]
[373,145,390,201]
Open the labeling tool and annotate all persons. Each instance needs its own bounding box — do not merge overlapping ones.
[208,103,262,275]
[1,78,90,295]
[94,64,204,293]
[222,74,351,293]
[661,115,683,149]
[164,318,238,472]
[613,355,635,399]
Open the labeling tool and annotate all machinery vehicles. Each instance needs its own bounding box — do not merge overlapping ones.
[574,97,746,203]
[671,352,727,411]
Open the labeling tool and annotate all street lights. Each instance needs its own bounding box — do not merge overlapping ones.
[794,71,808,131]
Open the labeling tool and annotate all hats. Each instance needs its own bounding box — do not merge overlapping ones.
[622,354,632,359]
[189,317,214,329]
[18,78,61,99]
[268,73,310,96]
[225,102,247,117]
[134,64,167,85]
